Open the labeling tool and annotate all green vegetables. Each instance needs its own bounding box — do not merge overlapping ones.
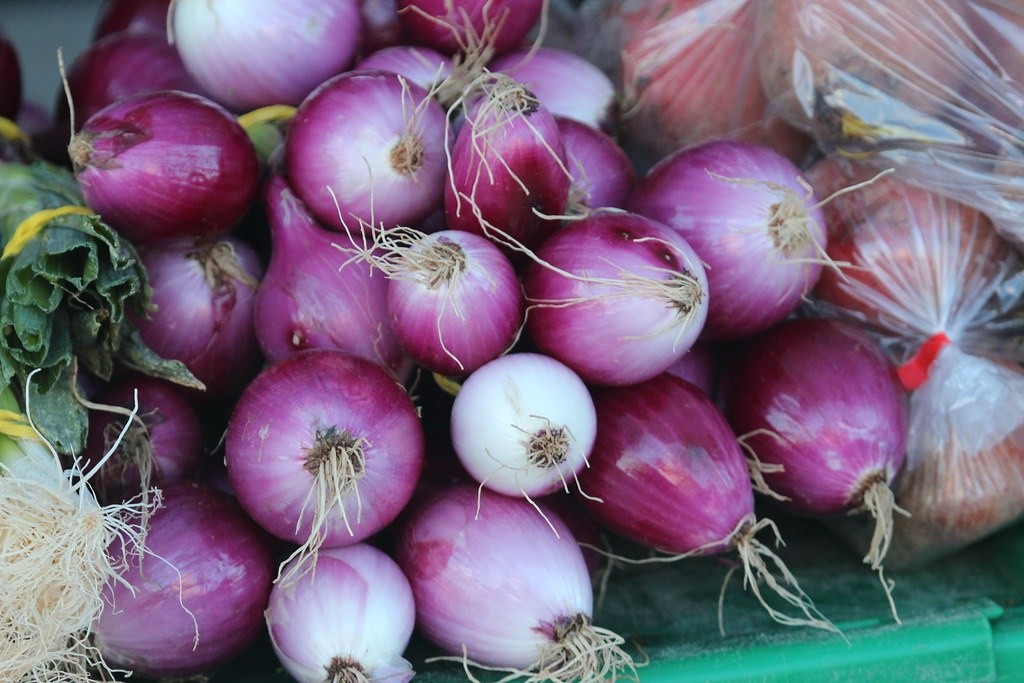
[1,118,204,683]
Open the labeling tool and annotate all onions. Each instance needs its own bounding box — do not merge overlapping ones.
[55,0,909,682]
